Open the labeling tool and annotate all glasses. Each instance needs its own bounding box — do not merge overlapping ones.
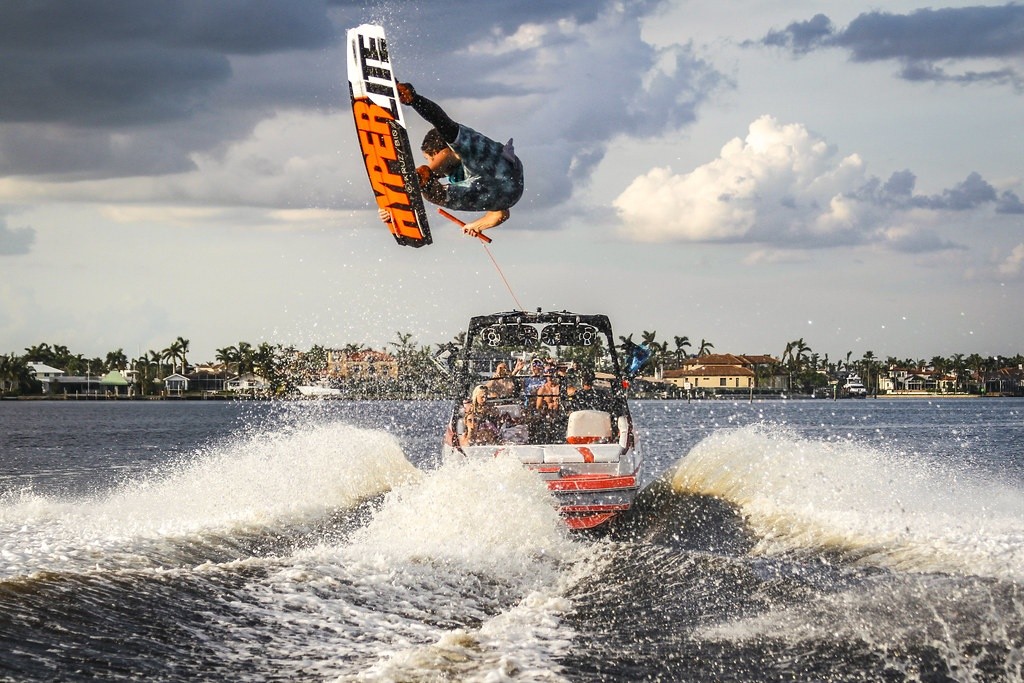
[480,386,488,389]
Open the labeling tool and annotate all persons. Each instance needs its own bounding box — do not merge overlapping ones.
[513,357,598,410]
[460,414,497,446]
[485,363,515,400]
[464,384,500,426]
[535,372,560,410]
[376,75,524,237]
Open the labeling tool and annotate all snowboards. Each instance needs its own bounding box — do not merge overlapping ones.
[342,22,437,248]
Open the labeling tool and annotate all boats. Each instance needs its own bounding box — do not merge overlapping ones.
[444,310,643,540]
